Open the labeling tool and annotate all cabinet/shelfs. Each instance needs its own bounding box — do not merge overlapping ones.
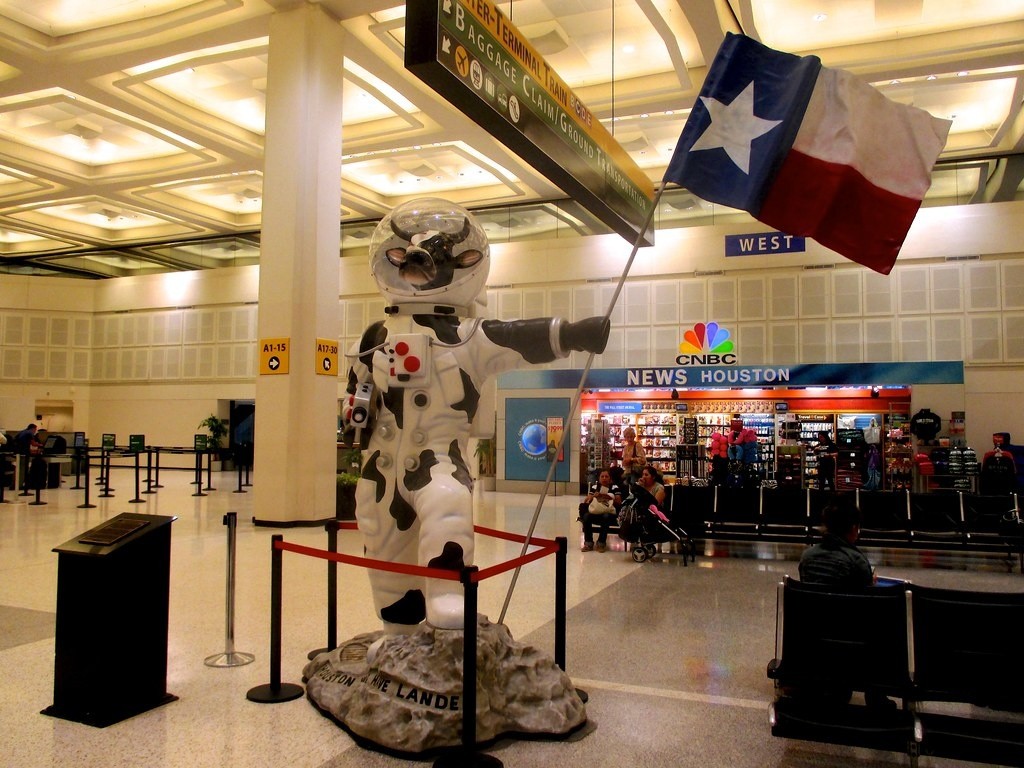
[581,409,914,486]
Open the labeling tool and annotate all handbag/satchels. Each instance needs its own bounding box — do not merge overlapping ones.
[631,442,648,478]
[588,493,617,514]
[862,417,885,491]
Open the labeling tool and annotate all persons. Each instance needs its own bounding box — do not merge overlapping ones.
[34,428,48,450]
[335,196,610,671]
[637,466,665,507]
[798,502,875,590]
[581,468,622,552]
[622,428,647,485]
[814,430,839,493]
[11,423,44,456]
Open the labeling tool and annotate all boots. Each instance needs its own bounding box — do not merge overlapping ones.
[580,541,594,552]
[595,541,607,553]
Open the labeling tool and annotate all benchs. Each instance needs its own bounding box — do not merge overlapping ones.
[768,574,1024,768]
[580,478,1023,574]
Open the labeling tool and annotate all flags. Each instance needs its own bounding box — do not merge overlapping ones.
[663,30,953,278]
